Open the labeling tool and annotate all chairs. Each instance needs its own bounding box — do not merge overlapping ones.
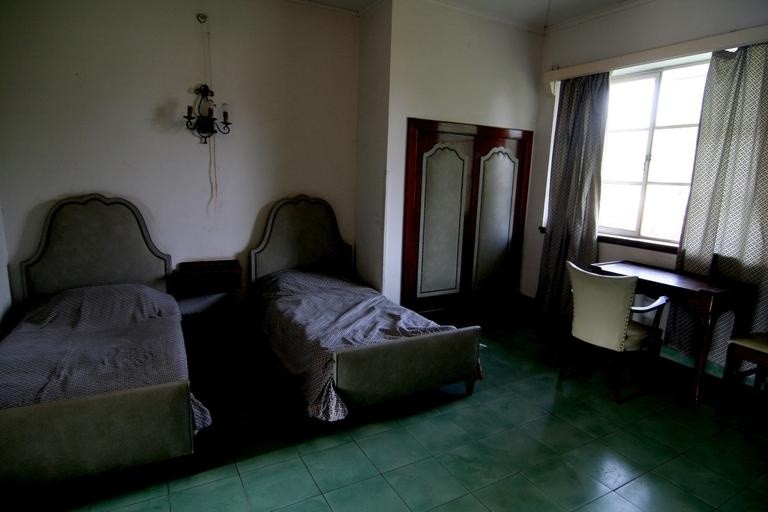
[565,259,671,390]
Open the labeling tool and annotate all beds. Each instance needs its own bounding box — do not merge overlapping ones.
[249,193,484,429]
[1,192,195,492]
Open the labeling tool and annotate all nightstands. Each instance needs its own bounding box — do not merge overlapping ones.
[174,260,242,372]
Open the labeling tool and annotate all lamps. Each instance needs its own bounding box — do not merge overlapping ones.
[184,82,233,143]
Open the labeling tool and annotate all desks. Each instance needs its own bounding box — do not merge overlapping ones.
[588,260,728,404]
